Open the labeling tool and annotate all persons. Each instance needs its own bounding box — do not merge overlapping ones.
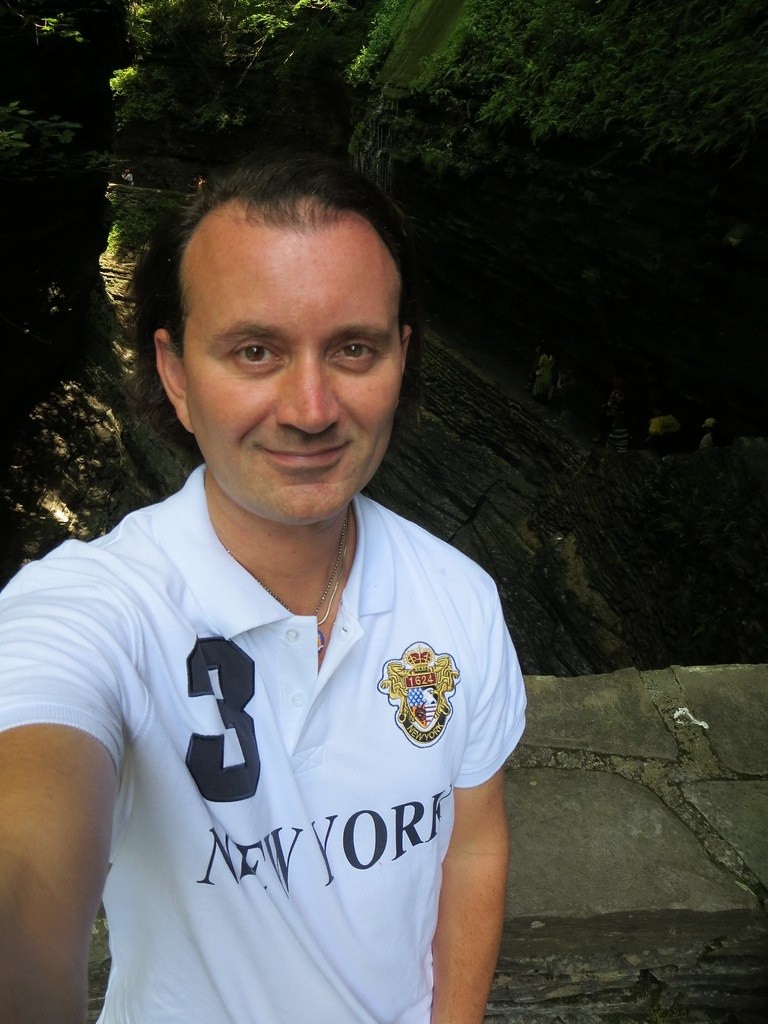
[1,151,529,1024]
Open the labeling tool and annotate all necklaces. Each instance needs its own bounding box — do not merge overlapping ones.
[217,503,349,654]
